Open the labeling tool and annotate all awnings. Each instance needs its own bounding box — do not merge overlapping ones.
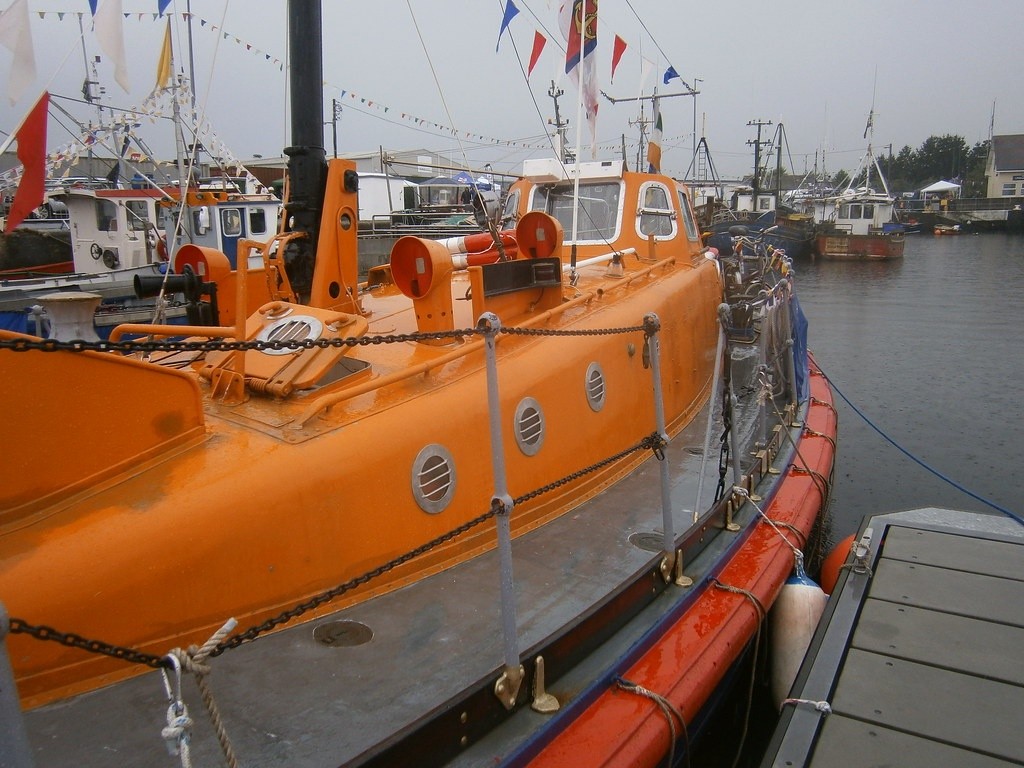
[419,172,491,190]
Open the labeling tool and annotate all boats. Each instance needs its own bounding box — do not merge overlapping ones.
[934,225,963,235]
[0,0,843,768]
[0,0,910,343]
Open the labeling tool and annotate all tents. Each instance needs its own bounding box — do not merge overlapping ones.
[920,180,961,206]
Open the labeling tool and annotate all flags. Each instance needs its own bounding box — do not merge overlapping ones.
[558,0,599,150]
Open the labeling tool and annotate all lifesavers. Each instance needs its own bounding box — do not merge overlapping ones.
[433,229,519,271]
[156,235,170,262]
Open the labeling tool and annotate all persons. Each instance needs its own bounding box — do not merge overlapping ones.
[461,187,470,204]
